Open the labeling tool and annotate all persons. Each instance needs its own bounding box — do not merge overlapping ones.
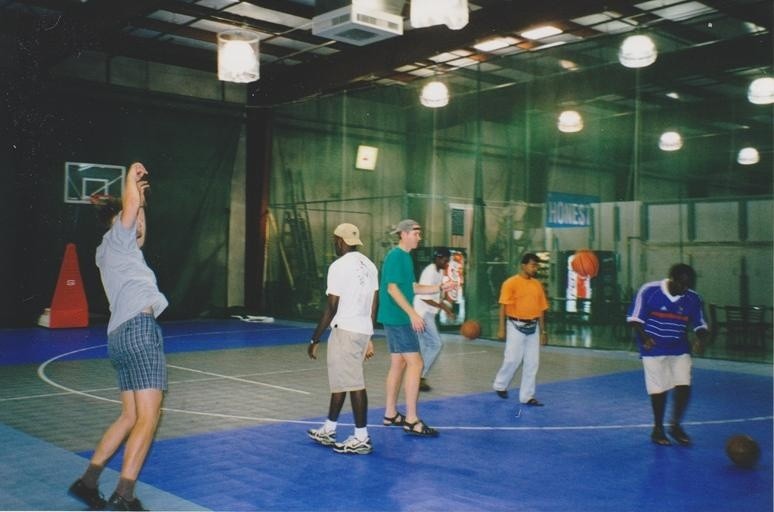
[413,245,457,391]
[305,221,380,455]
[625,263,710,447]
[491,253,549,407]
[374,218,440,439]
[65,159,169,511]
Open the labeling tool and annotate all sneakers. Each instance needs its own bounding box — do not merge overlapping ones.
[107,492,145,511]
[306,379,543,455]
[669,425,689,445]
[69,479,107,511]
[651,428,670,445]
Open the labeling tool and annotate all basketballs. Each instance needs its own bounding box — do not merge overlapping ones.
[727,436,761,469]
[573,252,598,276]
[462,320,480,338]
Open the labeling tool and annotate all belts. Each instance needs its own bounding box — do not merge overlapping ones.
[509,316,531,322]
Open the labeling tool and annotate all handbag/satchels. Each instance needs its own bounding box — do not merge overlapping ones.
[517,322,537,334]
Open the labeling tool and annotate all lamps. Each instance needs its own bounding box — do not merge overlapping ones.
[747,66,773,107]
[735,145,761,169]
[658,126,685,154]
[215,28,261,84]
[418,67,452,111]
[558,97,586,135]
[408,1,470,33]
[617,22,659,73]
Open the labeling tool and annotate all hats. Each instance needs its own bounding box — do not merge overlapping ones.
[390,220,421,236]
[334,223,362,246]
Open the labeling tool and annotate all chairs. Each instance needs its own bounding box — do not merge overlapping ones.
[545,294,637,344]
[709,305,770,353]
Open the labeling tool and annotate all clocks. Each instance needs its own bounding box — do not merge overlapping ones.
[355,143,380,171]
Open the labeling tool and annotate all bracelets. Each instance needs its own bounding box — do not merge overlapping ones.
[138,203,145,209]
[309,338,319,344]
[542,331,547,335]
[438,284,444,292]
